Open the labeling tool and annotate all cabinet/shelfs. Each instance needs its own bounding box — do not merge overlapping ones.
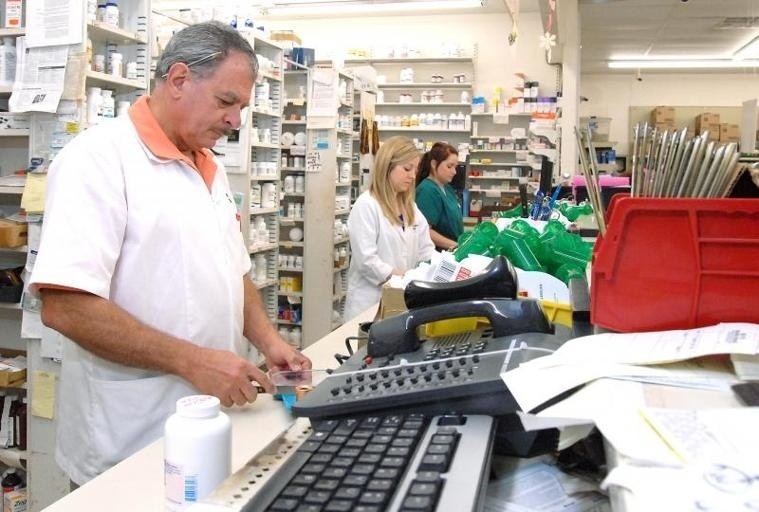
[381,55,619,244]
[0,0,279,507]
[279,50,379,352]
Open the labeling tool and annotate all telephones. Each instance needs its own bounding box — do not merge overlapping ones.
[291,298,573,458]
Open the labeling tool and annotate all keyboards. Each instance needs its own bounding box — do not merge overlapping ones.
[202,410,500,512]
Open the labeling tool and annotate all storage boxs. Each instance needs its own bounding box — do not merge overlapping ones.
[652,105,740,149]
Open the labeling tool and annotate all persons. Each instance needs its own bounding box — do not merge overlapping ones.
[28,21,311,492]
[343,136,439,323]
[416,141,464,252]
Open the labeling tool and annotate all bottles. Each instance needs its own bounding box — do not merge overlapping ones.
[84,0,138,119]
[376,65,594,285]
[163,393,231,505]
[250,46,352,352]
